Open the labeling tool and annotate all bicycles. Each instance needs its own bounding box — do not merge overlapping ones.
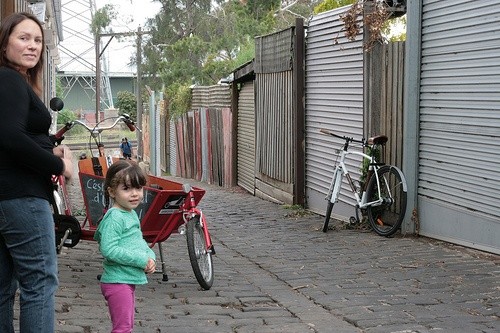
[319,129,408,235]
[47,97,215,290]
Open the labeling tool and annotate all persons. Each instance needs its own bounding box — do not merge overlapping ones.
[94,160,157,333]
[0,12,75,333]
[119,137,132,160]
